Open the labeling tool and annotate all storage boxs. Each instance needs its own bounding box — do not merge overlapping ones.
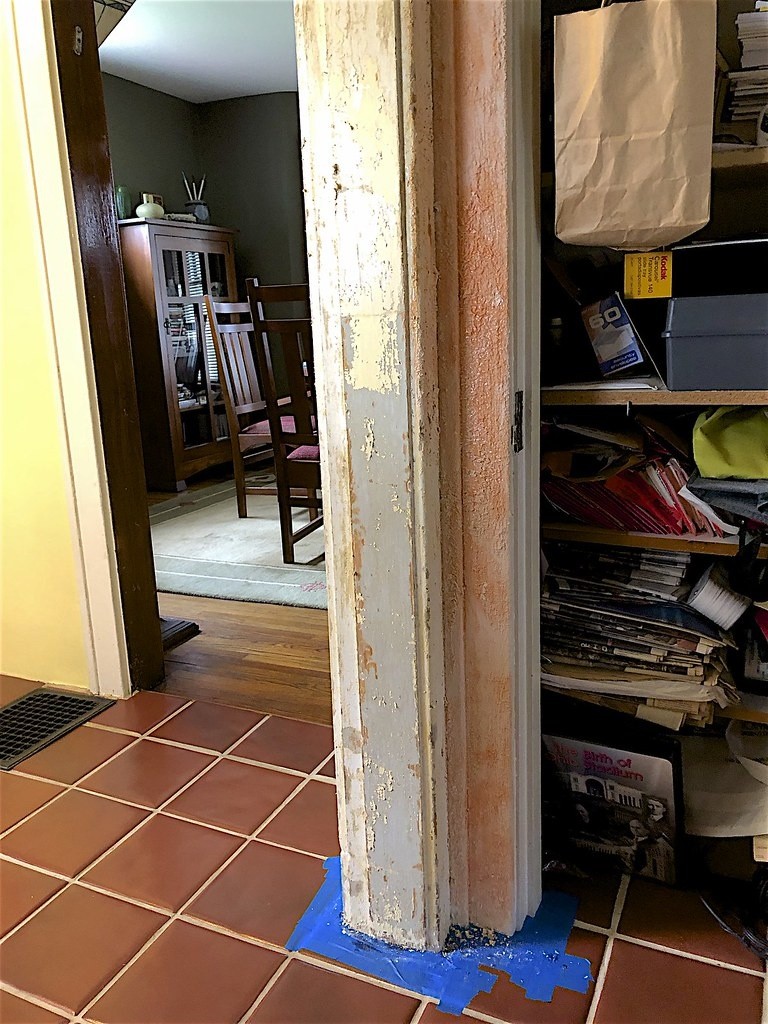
[660,292,768,390]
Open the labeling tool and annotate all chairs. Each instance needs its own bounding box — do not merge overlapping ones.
[247,287,324,565]
[202,293,322,522]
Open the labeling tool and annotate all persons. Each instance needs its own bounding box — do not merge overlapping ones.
[643,795,668,823]
[628,819,655,869]
[573,803,614,845]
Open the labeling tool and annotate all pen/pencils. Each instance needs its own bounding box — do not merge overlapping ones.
[197,173,206,199]
[182,171,193,201]
[191,175,197,201]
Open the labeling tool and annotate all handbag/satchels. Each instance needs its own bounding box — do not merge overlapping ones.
[682,720,768,837]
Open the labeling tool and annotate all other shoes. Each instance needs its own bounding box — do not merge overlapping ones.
[553,0,727,254]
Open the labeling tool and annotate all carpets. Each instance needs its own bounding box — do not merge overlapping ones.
[148,467,327,612]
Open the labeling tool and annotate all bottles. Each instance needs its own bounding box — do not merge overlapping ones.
[547,318,564,346]
[116,186,131,220]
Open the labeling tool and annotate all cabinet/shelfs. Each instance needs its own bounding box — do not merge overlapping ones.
[523,133,768,739]
[119,217,251,494]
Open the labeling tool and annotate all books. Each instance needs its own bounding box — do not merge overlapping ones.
[536,459,729,538]
[535,548,725,724]
